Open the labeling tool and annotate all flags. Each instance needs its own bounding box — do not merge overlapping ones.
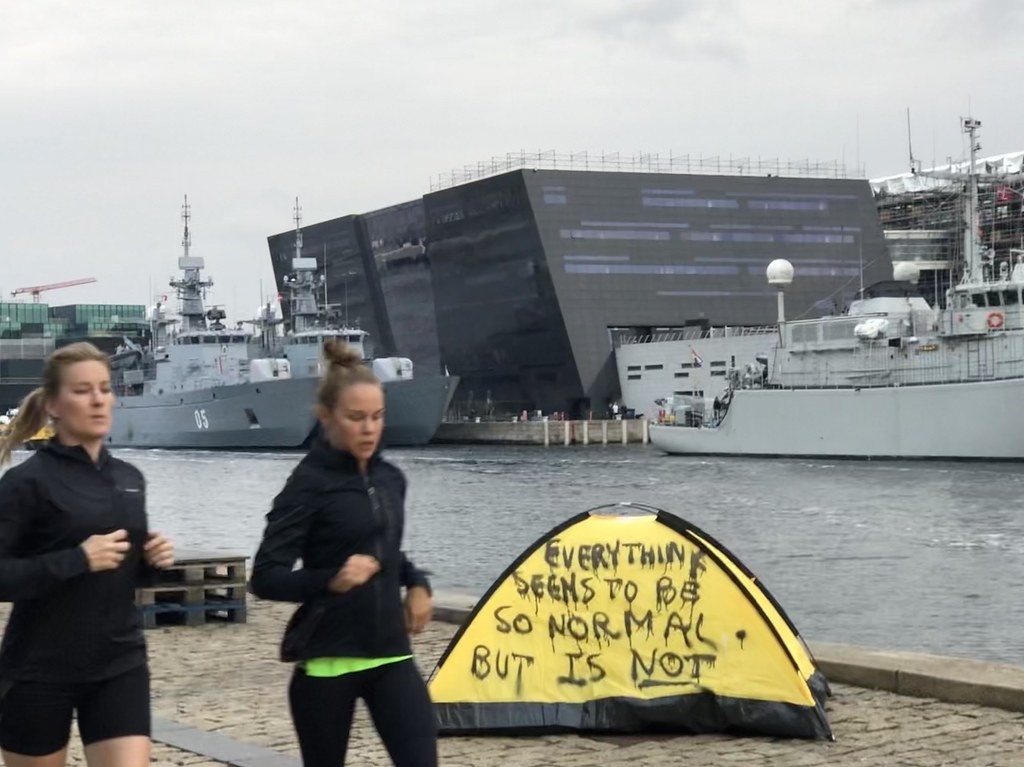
[692,350,703,368]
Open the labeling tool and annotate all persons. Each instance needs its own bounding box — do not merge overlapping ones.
[251,340,437,767]
[0,342,174,767]
[607,401,619,419]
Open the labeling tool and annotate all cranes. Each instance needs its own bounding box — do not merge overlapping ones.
[10,278,97,303]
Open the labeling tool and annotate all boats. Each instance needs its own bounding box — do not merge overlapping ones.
[0,408,56,450]
[649,117,1024,459]
[101,195,461,449]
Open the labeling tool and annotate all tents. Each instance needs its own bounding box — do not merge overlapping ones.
[426,503,834,738]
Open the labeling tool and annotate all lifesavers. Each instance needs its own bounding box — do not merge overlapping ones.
[987,311,1003,329]
[658,409,665,423]
